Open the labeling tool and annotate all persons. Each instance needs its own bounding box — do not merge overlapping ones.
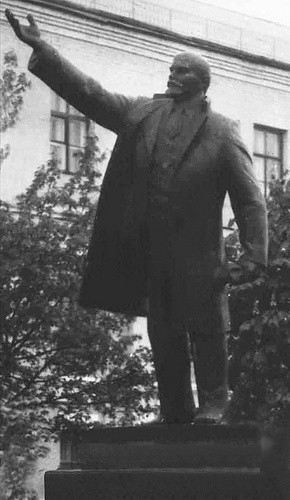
[5,8,268,425]
